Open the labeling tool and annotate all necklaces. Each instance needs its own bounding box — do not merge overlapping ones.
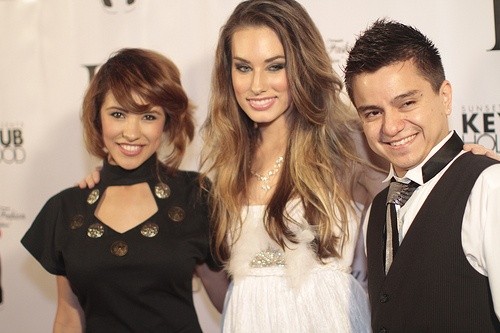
[247,151,284,190]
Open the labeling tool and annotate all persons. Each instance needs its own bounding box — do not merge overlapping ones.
[345,17,500,333]
[74,0,500,333]
[21,48,234,333]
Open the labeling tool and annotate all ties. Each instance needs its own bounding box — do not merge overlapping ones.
[385,130,465,278]
